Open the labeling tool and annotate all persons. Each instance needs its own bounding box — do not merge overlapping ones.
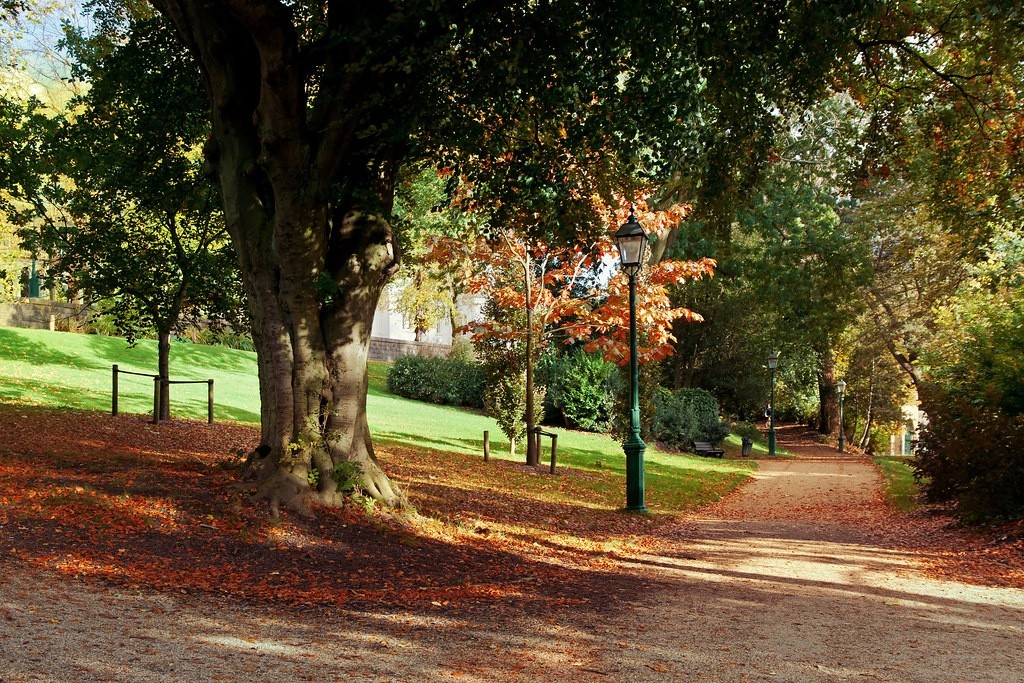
[764,404,772,428]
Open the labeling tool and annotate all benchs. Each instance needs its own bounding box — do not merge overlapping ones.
[691,441,724,459]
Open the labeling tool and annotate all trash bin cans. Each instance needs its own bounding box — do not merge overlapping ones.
[741,438,753,457]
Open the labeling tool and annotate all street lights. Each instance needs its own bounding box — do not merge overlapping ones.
[836,378,847,451]
[766,351,779,456]
[612,200,650,514]
